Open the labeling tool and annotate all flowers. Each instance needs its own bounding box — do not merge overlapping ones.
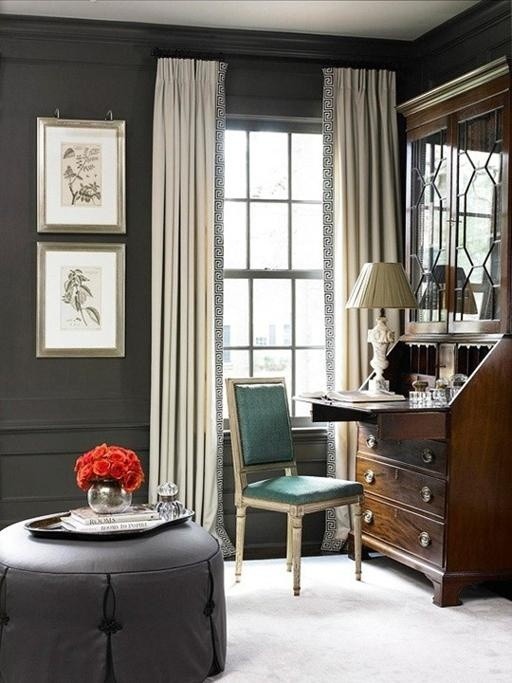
[73,443,145,494]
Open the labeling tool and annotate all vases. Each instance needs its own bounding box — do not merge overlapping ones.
[87,479,133,515]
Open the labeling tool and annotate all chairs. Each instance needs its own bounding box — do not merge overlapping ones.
[224,377,364,596]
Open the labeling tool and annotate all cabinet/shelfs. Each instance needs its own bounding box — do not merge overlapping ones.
[290,54,512,608]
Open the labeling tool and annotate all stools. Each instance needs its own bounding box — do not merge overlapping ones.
[0,511,227,683]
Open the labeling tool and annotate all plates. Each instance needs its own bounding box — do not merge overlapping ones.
[24,504,195,541]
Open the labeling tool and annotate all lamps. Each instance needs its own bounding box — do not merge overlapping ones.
[345,263,418,398]
[418,265,479,316]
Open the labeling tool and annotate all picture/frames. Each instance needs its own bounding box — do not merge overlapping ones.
[35,242,126,359]
[36,117,126,234]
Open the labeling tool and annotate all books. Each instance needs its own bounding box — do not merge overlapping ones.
[297,389,406,403]
[60,507,168,533]
